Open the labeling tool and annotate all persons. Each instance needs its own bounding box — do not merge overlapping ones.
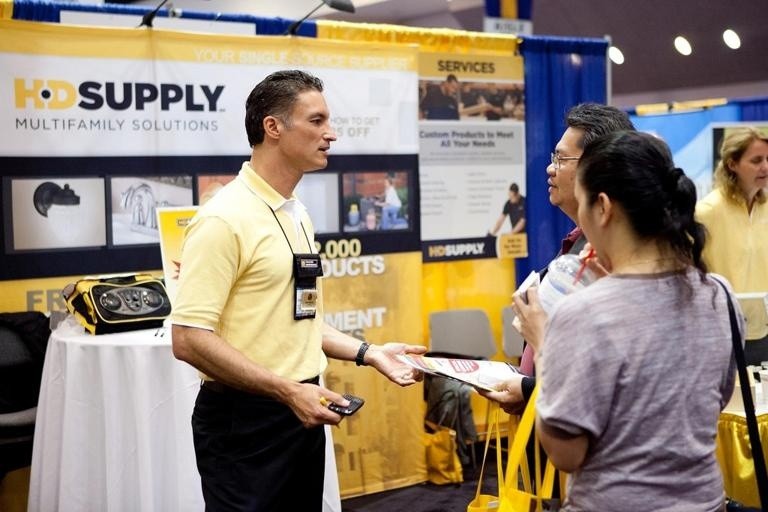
[492,183,526,234]
[475,103,636,415]
[684,127,768,381]
[419,74,525,121]
[374,176,407,230]
[170,70,427,512]
[509,129,748,512]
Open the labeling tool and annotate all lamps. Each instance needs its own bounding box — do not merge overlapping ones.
[288,0,354,36]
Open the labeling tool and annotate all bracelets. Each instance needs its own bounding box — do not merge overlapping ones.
[356,342,371,366]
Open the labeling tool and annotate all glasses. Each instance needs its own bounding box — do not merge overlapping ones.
[551,152,579,170]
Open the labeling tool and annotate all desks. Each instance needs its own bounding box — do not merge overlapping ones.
[28,294,341,512]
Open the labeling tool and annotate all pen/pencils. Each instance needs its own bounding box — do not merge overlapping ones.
[155,329,159,336]
[161,332,164,337]
[320,397,342,430]
[572,247,594,285]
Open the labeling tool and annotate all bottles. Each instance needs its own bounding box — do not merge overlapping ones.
[348,203,361,226]
[366,208,376,231]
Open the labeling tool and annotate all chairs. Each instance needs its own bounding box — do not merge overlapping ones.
[432,309,513,469]
[501,307,527,363]
[1,311,50,479]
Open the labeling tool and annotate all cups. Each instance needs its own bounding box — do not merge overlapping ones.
[758,369,767,406]
[537,254,594,330]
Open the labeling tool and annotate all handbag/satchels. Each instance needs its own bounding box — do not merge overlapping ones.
[467,492,557,512]
[423,420,464,485]
[61,273,172,336]
